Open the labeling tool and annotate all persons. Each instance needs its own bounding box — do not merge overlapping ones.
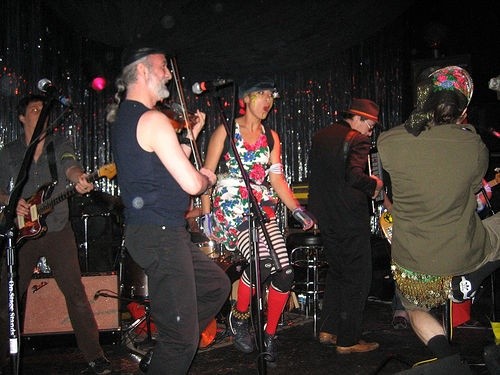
[308,97,384,354]
[115,41,231,375]
[201,70,314,361]
[0,94,113,375]
[376,65,500,360]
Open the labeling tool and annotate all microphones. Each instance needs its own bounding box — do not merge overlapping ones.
[192,79,233,94]
[94,291,100,300]
[37,79,71,106]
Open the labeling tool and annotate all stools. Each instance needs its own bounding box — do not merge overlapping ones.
[288,232,327,335]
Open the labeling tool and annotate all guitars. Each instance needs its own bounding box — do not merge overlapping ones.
[13,161,117,249]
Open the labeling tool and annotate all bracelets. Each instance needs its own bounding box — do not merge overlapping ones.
[180,137,194,146]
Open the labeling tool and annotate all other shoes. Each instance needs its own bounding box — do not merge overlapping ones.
[336,339,380,354]
[87,355,112,375]
[483,344,500,375]
[320,332,339,345]
[139,349,154,372]
[262,324,278,366]
[0,358,22,375]
[396,357,472,375]
[228,302,253,353]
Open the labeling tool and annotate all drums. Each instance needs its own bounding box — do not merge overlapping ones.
[194,240,244,266]
[122,241,149,301]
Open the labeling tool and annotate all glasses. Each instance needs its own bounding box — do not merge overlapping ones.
[362,117,374,132]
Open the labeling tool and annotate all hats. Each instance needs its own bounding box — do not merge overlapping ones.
[122,42,170,67]
[342,99,380,124]
[237,66,276,99]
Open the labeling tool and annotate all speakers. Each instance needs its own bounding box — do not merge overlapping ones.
[21,273,122,351]
[399,354,472,375]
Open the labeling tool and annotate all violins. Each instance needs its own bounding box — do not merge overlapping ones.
[155,101,198,131]
